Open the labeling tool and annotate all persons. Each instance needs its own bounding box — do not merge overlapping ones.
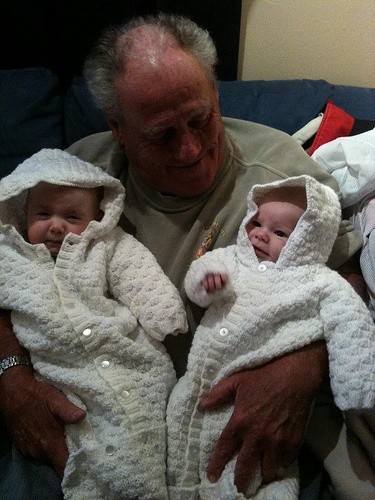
[0,13,370,500]
[0,147,188,500]
[166,174,375,500]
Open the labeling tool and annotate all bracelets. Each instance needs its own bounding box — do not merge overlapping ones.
[0,355,32,375]
